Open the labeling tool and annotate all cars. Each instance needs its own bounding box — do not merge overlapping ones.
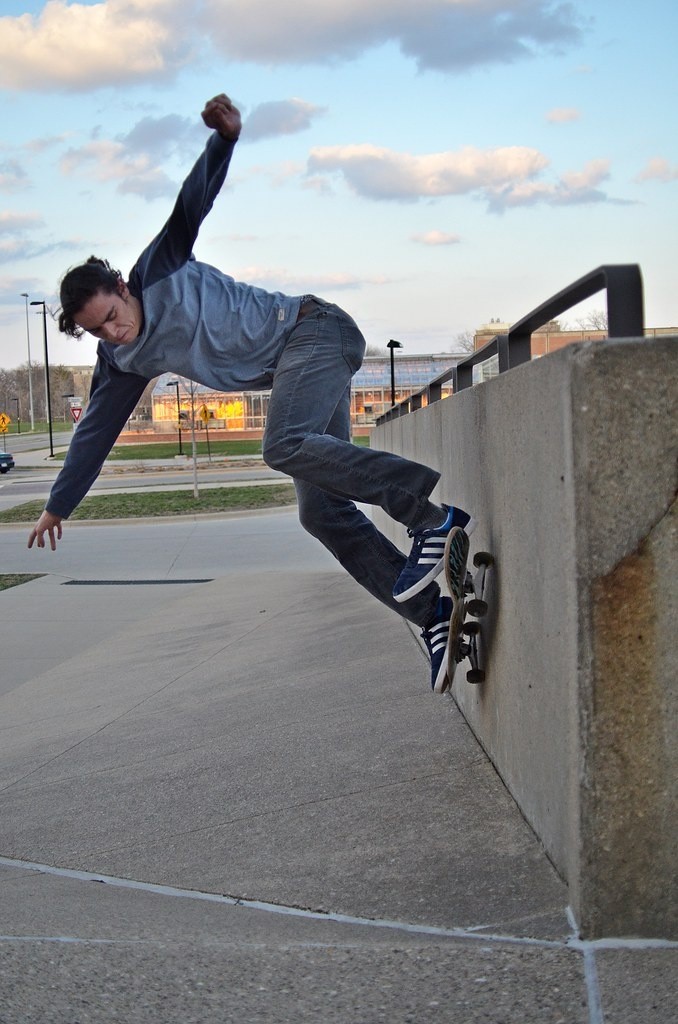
[0,451,15,474]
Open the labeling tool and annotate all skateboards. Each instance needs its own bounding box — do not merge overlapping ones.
[443,525,496,693]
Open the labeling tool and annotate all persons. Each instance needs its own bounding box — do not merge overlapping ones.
[27,94,479,695]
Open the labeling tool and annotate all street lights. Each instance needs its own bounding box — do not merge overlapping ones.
[19,293,36,430]
[62,393,76,423]
[166,381,183,456]
[30,300,55,458]
[10,397,21,433]
[386,339,403,407]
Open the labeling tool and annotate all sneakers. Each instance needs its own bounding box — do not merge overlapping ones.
[392,502,478,604]
[419,596,454,695]
